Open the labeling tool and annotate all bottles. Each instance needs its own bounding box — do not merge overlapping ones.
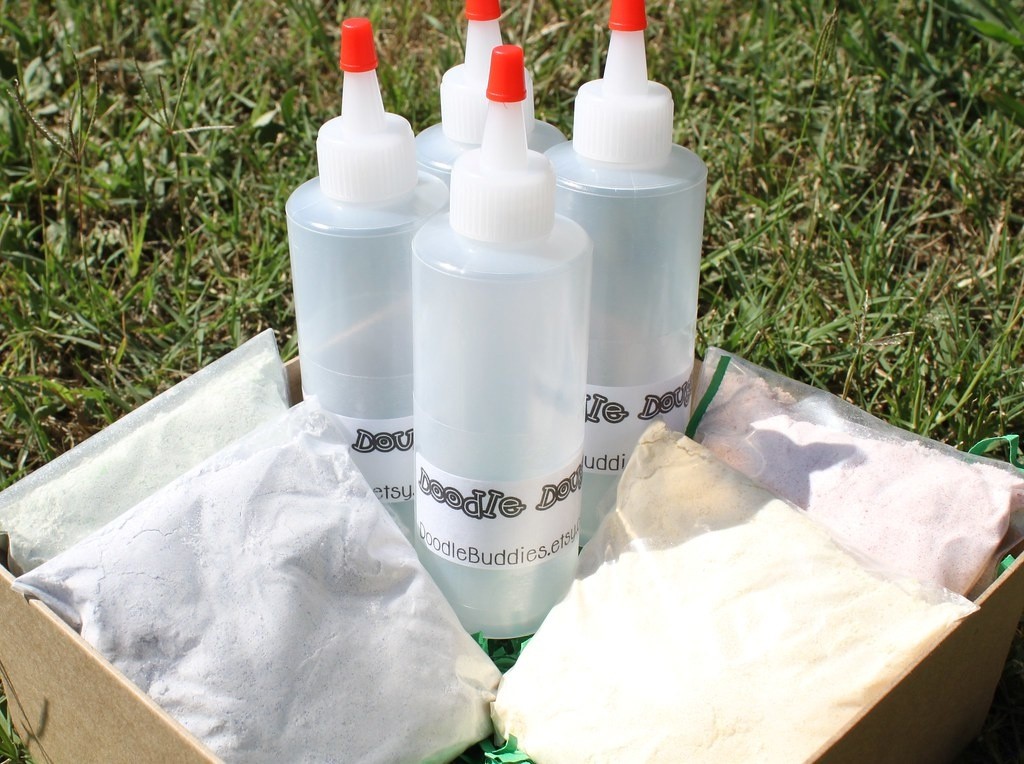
[544,0,706,547]
[413,0,566,208]
[285,18,450,531]
[409,45,592,637]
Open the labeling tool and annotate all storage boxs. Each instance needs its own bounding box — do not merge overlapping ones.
[0,353,1024,764]
